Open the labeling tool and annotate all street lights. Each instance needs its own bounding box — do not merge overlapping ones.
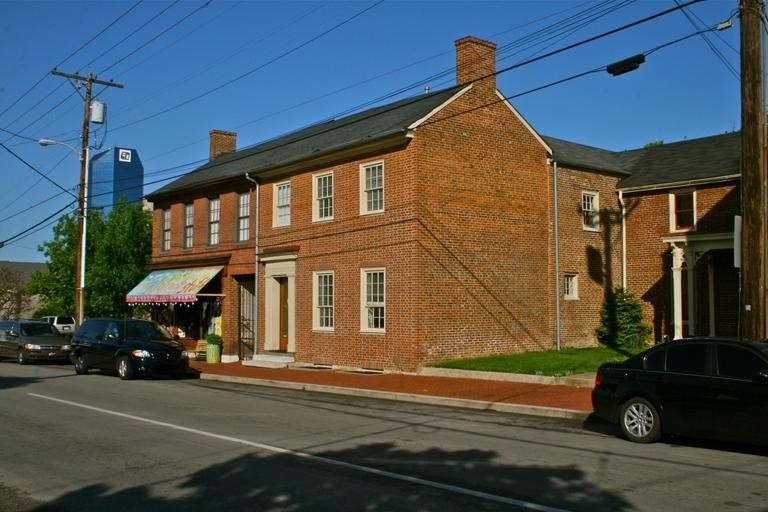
[39,138,89,332]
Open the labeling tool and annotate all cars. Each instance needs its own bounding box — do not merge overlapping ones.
[0,315,189,380]
[593,336,768,443]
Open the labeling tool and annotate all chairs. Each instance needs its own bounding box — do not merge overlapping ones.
[194,339,207,360]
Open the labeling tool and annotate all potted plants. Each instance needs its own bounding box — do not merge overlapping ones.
[206,333,222,364]
